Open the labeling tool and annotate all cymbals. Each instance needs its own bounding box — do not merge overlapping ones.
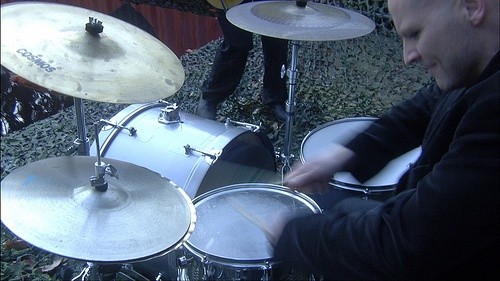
[0,155,198,266]
[1,0,185,105]
[225,0,376,41]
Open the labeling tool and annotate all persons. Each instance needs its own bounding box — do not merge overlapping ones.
[196,0,297,125]
[263,0,500,281]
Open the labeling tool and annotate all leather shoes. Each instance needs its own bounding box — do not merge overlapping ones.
[269,104,295,125]
[196,96,219,120]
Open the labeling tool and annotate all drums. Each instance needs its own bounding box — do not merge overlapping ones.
[88,104,278,200]
[300,116,422,201]
[175,182,323,280]
